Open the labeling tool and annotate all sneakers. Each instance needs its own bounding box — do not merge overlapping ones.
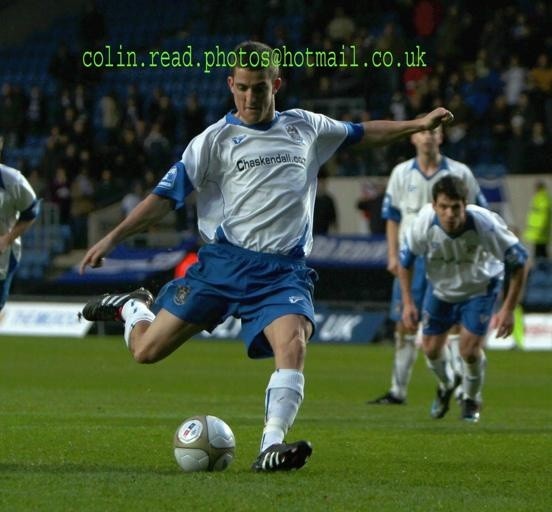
[364,390,408,406]
[251,439,313,473]
[430,372,463,419]
[83,287,155,322]
[461,392,483,423]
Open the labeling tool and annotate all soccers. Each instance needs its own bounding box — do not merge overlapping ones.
[174,414,235,472]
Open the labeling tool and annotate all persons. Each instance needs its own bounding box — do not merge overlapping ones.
[0,0,552,222]
[0,163,42,317]
[73,41,456,474]
[364,113,489,407]
[398,174,532,424]
